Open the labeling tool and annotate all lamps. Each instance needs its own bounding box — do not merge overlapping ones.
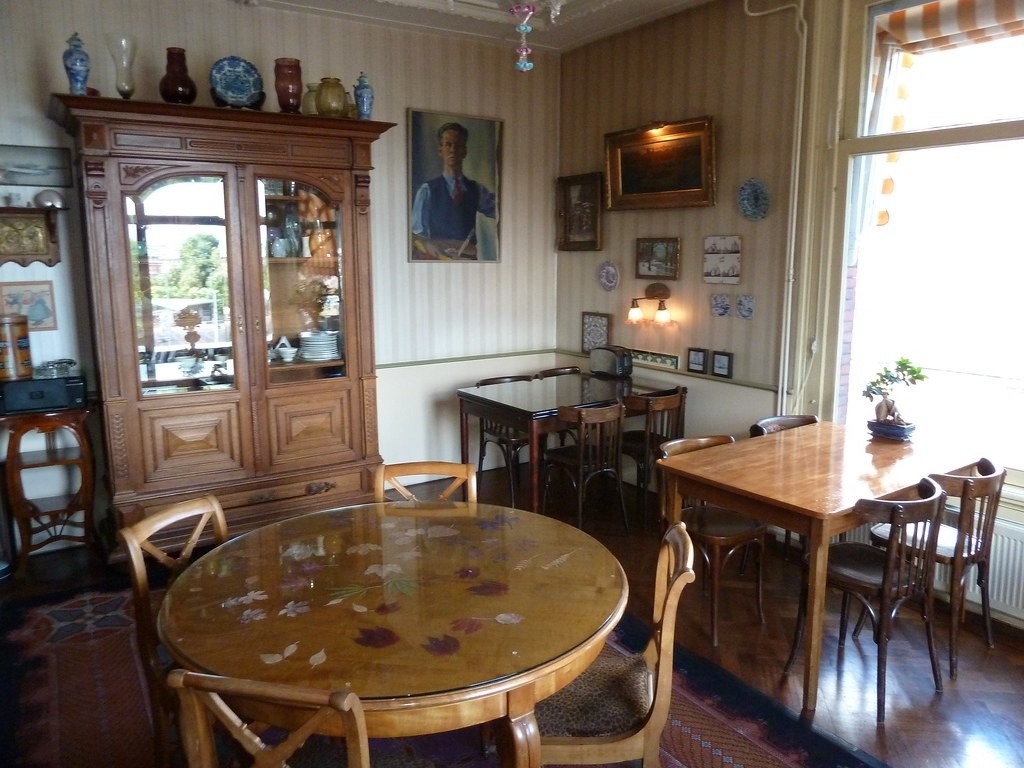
[625,282,674,326]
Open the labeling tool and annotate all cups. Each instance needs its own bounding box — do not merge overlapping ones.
[274,57,301,113]
[105,34,137,98]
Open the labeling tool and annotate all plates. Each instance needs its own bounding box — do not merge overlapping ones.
[297,331,342,362]
[209,55,263,107]
[598,261,619,291]
[736,177,770,221]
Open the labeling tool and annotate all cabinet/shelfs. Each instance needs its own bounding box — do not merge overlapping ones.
[45,91,399,585]
[0,400,101,598]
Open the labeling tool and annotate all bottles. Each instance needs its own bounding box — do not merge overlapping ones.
[160,47,197,105]
[267,182,336,259]
[354,75,374,120]
[301,82,319,115]
[316,78,347,117]
[63,35,90,96]
[0,315,32,382]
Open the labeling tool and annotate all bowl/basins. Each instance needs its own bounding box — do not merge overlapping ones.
[279,347,297,362]
[214,354,230,364]
[176,356,196,371]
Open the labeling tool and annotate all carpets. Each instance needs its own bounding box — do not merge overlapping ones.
[0,574,891,768]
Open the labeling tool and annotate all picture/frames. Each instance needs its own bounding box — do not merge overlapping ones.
[712,351,735,379]
[687,346,709,374]
[581,310,612,355]
[557,171,602,250]
[0,145,73,188]
[603,114,716,211]
[635,238,680,282]
[0,281,58,331]
[406,106,506,264]
[629,349,678,370]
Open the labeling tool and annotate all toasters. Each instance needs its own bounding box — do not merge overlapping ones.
[589,345,633,378]
[590,375,632,402]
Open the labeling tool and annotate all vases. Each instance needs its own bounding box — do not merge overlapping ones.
[159,47,197,105]
[273,57,347,118]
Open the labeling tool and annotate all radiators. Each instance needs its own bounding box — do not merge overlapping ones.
[767,502,1024,631]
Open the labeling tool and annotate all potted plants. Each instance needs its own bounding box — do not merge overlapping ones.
[862,356,930,442]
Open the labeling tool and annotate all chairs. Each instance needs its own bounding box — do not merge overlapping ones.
[478,522,696,768]
[117,492,371,768]
[476,365,688,539]
[374,462,477,503]
[658,414,1008,725]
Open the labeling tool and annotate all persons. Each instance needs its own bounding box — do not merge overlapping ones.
[317,276,340,331]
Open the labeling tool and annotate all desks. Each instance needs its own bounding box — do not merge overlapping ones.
[157,501,629,761]
[655,421,994,712]
[456,372,688,515]
